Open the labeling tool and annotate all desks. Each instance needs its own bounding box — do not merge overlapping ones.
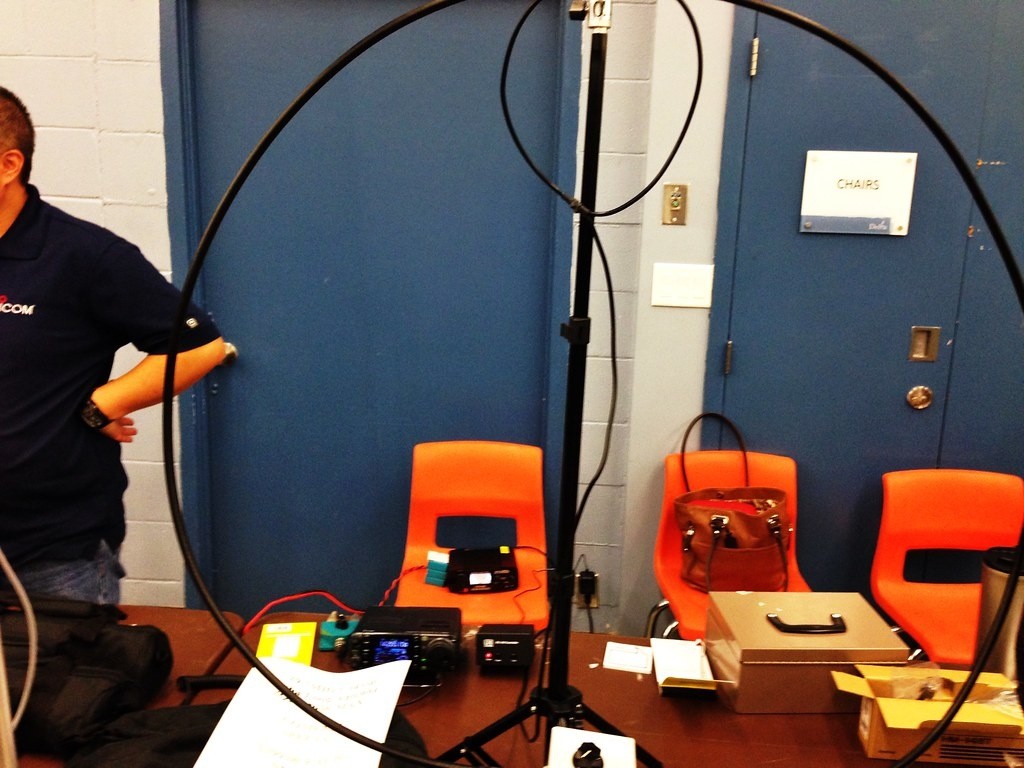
[189,613,999,768]
[0,606,244,768]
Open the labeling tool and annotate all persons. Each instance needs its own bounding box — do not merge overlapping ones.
[0,84,225,606]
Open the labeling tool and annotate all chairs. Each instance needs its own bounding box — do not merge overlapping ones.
[643,451,813,641]
[870,470,1024,663]
[394,442,550,631]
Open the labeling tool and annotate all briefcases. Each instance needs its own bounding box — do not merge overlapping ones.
[705,590,911,715]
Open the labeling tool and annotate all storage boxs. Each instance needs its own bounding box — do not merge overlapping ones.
[830,664,1024,766]
[704,591,910,713]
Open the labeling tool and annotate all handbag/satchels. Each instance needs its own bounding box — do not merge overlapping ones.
[674,413,793,595]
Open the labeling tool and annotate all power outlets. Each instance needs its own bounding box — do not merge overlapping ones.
[663,185,686,224]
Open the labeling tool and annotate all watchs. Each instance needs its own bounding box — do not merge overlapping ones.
[82,398,115,430]
